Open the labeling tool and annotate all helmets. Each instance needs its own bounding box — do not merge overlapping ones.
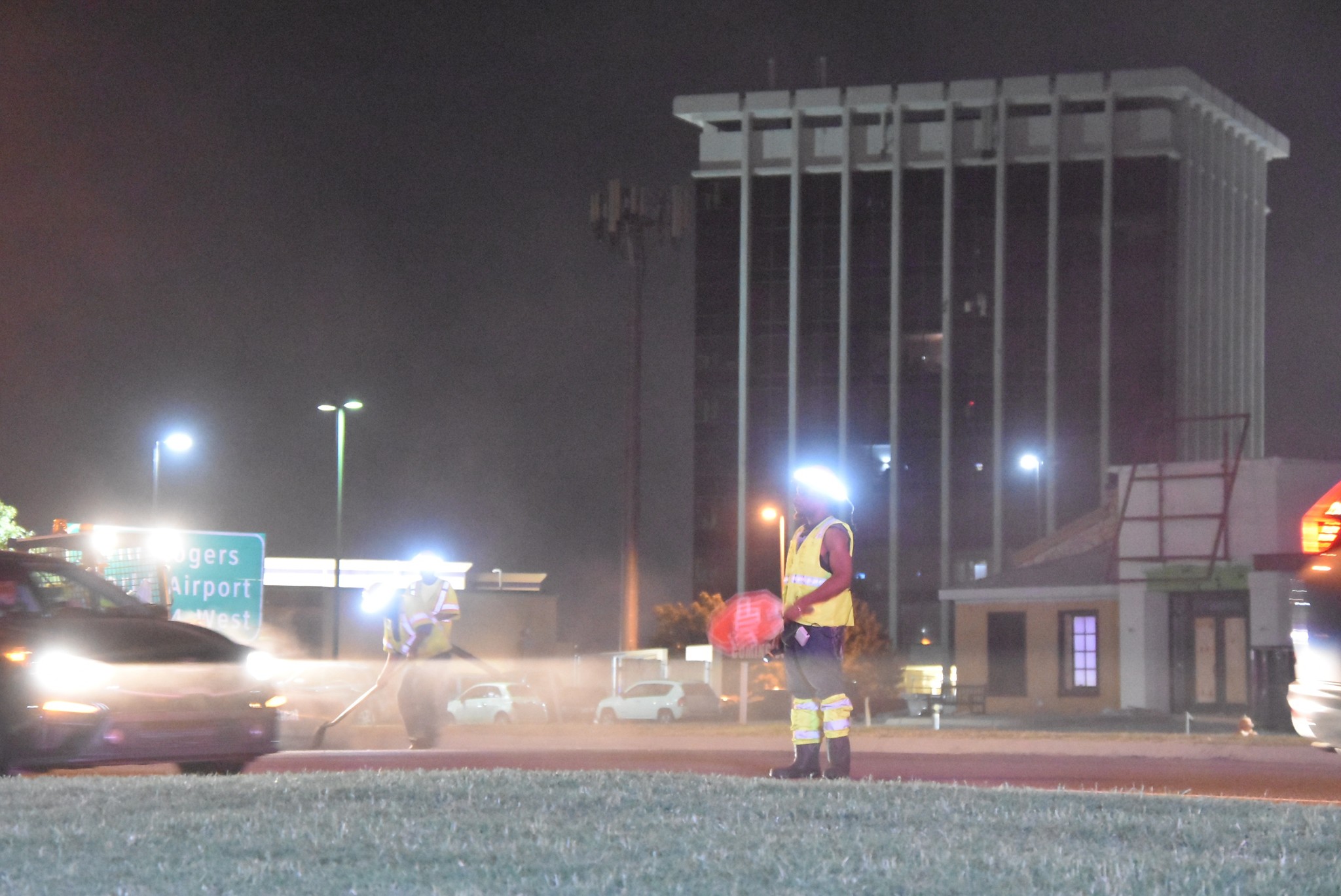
[413,552,445,572]
[359,584,395,614]
[793,464,847,502]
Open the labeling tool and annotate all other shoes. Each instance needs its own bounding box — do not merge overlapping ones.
[408,735,438,751]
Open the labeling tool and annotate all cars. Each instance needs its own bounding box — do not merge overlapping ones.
[447,682,548,729]
[1287,480,1341,753]
[0,549,279,776]
[596,678,719,724]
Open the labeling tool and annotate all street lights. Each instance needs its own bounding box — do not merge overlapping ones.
[317,399,363,659]
[761,506,785,601]
[150,430,193,511]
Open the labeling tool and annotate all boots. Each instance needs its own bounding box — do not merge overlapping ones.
[823,735,850,778]
[769,742,821,778]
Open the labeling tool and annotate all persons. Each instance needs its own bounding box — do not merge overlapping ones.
[770,465,857,779]
[382,552,460,751]
